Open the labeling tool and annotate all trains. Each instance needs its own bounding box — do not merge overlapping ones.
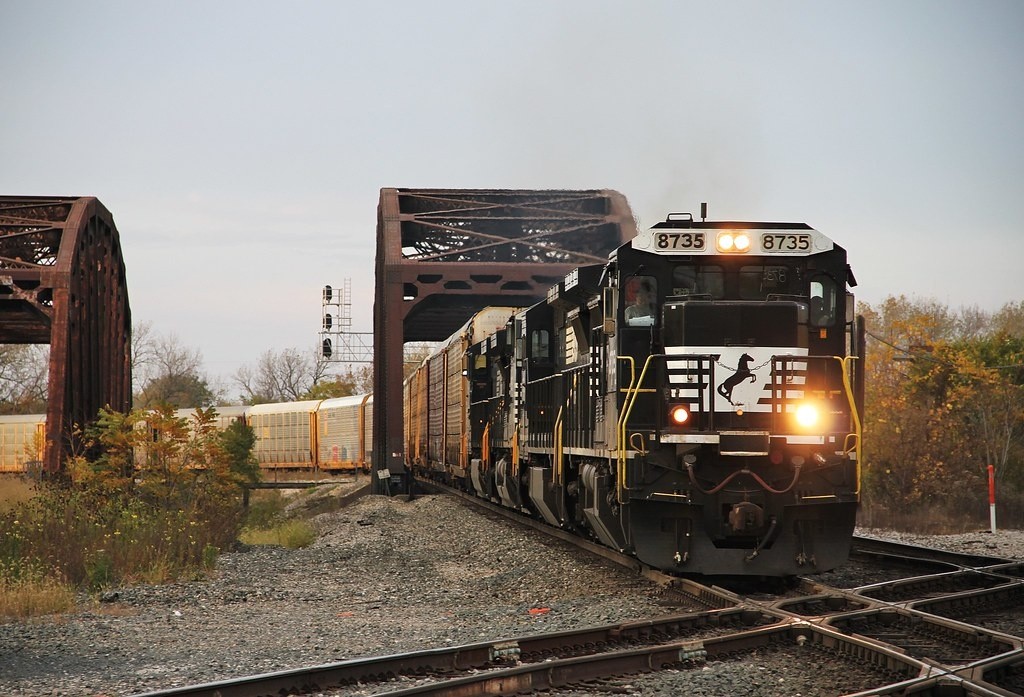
[0,204,868,582]
